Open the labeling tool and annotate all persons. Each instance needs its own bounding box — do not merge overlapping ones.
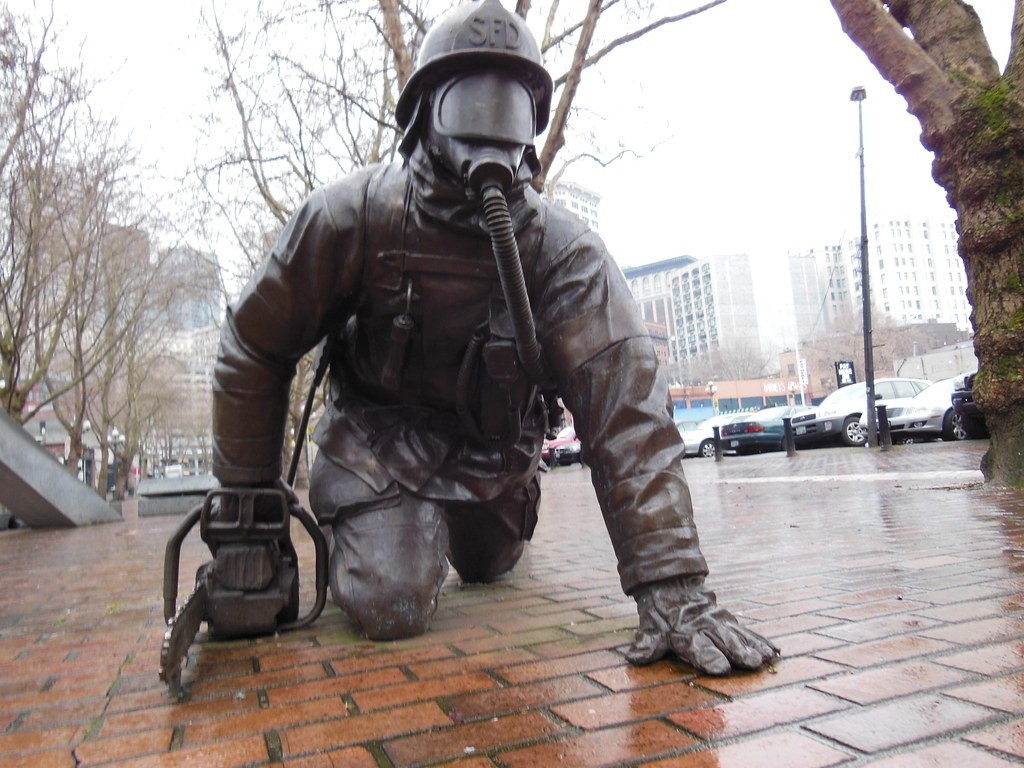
[212,0,781,676]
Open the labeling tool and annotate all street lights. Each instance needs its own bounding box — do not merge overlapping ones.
[106,425,126,480]
[849,85,878,449]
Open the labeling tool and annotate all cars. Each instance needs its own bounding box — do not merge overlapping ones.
[674,369,989,457]
[541,421,582,465]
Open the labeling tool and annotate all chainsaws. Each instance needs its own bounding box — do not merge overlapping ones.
[158,490,326,695]
